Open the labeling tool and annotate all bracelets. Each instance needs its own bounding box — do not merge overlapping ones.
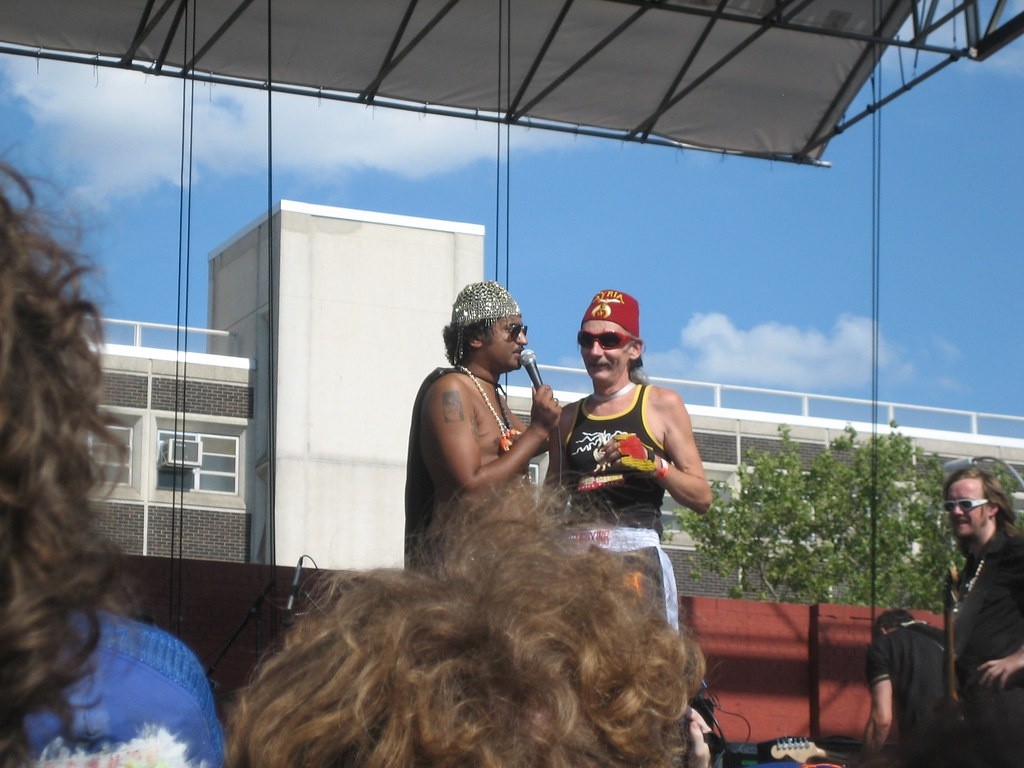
[652,459,668,480]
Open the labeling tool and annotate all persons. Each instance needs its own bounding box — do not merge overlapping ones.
[861,608,957,763]
[225,496,710,766]
[543,290,713,634]
[944,464,1024,768]
[404,281,562,574]
[0,159,225,768]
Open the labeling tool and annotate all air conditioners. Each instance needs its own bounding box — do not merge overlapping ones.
[160,439,203,469]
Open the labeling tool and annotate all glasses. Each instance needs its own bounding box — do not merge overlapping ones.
[578,330,636,350]
[943,498,989,512]
[492,324,527,340]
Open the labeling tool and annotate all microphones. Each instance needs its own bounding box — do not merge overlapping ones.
[519,350,544,393]
[286,558,304,612]
[206,679,220,692]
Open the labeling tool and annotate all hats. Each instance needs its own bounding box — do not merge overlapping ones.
[451,280,521,328]
[582,289,639,338]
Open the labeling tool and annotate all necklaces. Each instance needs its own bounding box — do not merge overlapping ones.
[967,560,984,592]
[457,364,521,454]
